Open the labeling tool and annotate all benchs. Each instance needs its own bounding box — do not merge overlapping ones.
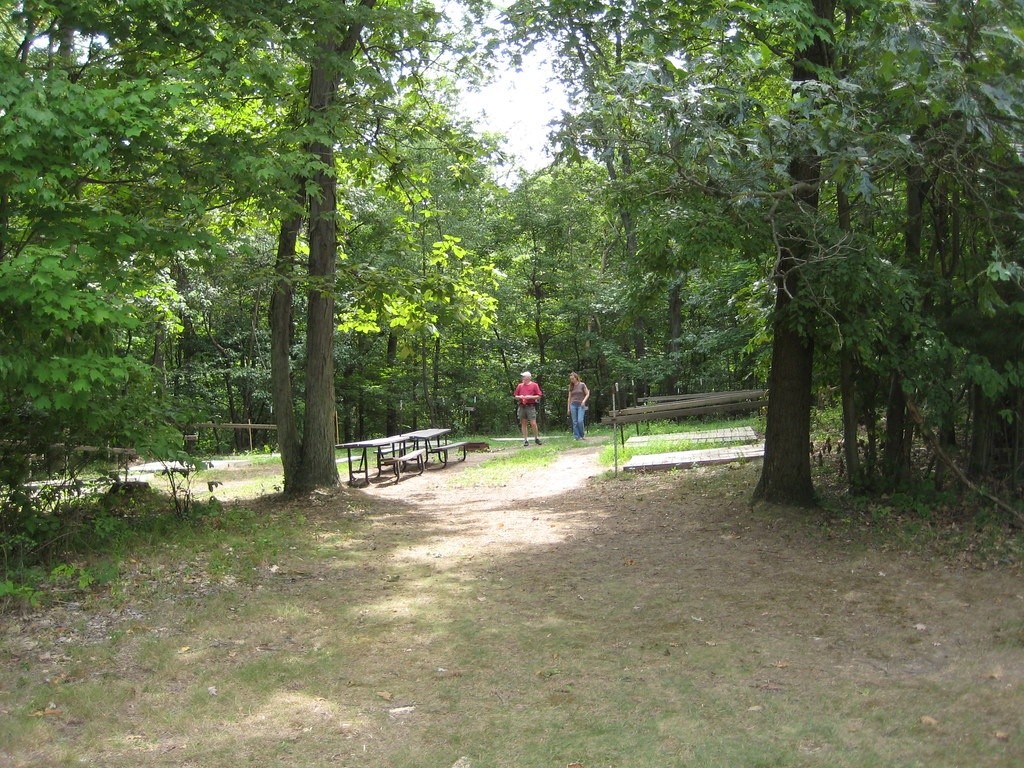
[373,442,467,484]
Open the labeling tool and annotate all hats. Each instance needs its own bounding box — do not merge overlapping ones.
[520,371,531,377]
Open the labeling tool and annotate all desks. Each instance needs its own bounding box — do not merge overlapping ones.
[401,428,452,471]
[334,436,410,486]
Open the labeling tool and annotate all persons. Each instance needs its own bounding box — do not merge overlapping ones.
[568,372,589,441]
[514,371,542,447]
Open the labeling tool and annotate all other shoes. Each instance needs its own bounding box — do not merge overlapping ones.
[535,439,542,445]
[524,442,529,446]
[581,436,585,440]
